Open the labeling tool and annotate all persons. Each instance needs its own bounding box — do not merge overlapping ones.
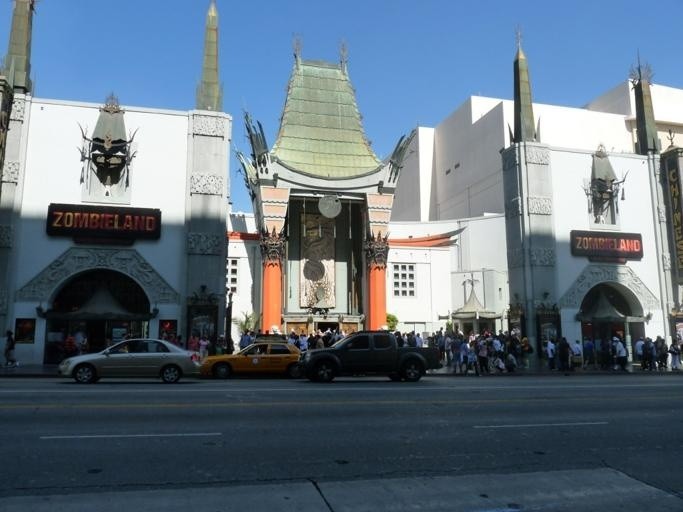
[168,334,234,360]
[119,334,129,353]
[542,336,597,371]
[394,328,528,377]
[610,335,683,373]
[4,331,17,367]
[239,328,345,351]
[65,327,87,357]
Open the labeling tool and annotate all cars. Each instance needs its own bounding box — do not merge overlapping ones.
[200,342,302,379]
[58,338,201,384]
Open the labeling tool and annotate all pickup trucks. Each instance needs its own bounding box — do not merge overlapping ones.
[299,331,443,382]
